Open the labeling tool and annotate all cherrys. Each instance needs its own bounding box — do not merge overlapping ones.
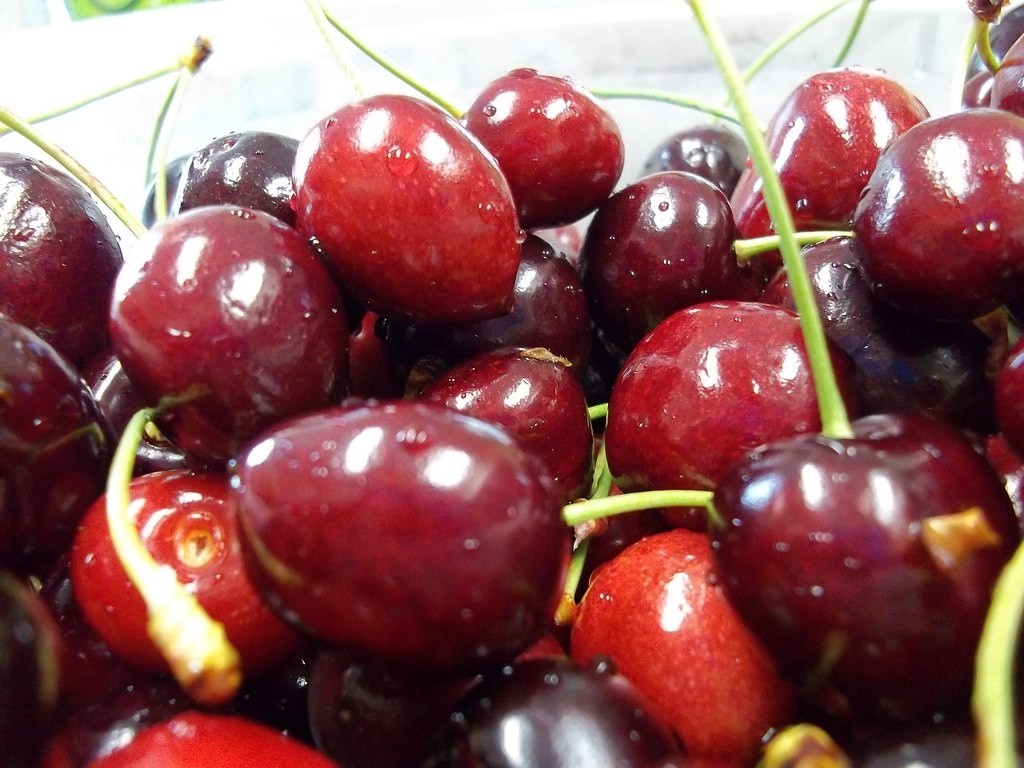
[0,0,1024,768]
